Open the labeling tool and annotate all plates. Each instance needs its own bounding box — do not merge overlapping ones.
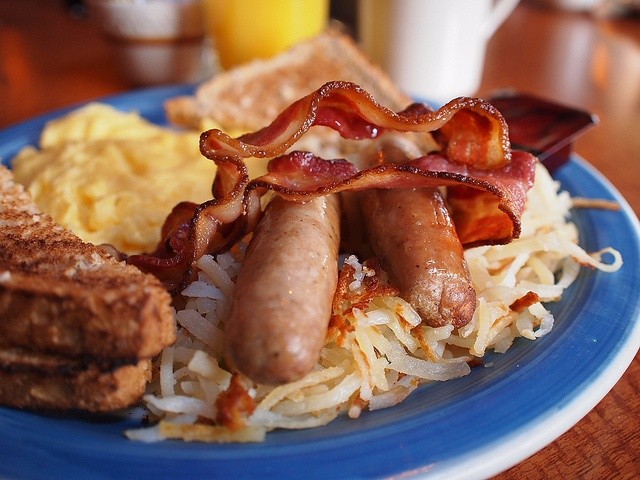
[2,74,639,474]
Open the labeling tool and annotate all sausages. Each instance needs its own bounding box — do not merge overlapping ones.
[362,186,475,323]
[223,191,341,382]
